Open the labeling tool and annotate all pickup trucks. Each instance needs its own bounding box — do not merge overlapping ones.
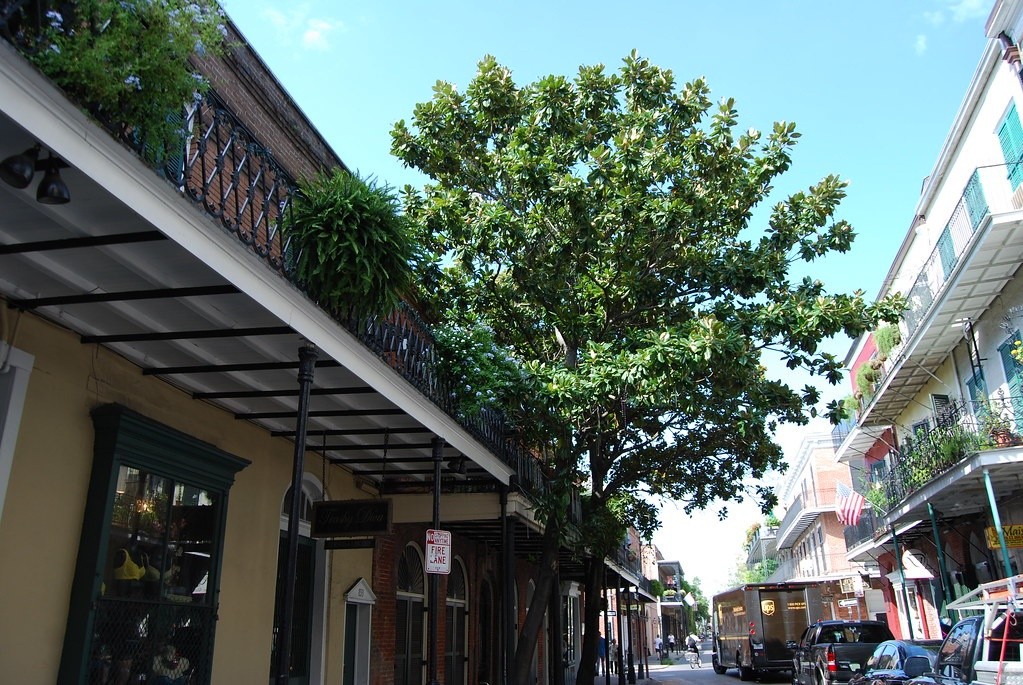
[784,619,900,685]
[902,601,1023,685]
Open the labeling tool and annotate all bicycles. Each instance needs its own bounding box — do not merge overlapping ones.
[688,640,702,669]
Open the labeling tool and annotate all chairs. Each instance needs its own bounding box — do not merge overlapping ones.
[821,630,838,643]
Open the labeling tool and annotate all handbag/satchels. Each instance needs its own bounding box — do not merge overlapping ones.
[688,637,696,647]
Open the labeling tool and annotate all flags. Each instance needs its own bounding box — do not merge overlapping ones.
[834,484,866,525]
[684,593,699,611]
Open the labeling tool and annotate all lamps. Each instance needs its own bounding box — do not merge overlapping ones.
[0,143,71,204]
[448,453,467,474]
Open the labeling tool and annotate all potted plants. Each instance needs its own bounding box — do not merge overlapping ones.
[844,325,901,409]
[976,391,1012,448]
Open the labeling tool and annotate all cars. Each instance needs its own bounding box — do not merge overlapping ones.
[846,638,944,685]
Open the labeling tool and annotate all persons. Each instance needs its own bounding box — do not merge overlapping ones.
[595,631,704,678]
[98,507,181,685]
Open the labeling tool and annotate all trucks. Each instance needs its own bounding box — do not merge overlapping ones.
[705,580,824,682]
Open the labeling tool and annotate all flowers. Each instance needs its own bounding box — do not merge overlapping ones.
[1010,340,1023,366]
